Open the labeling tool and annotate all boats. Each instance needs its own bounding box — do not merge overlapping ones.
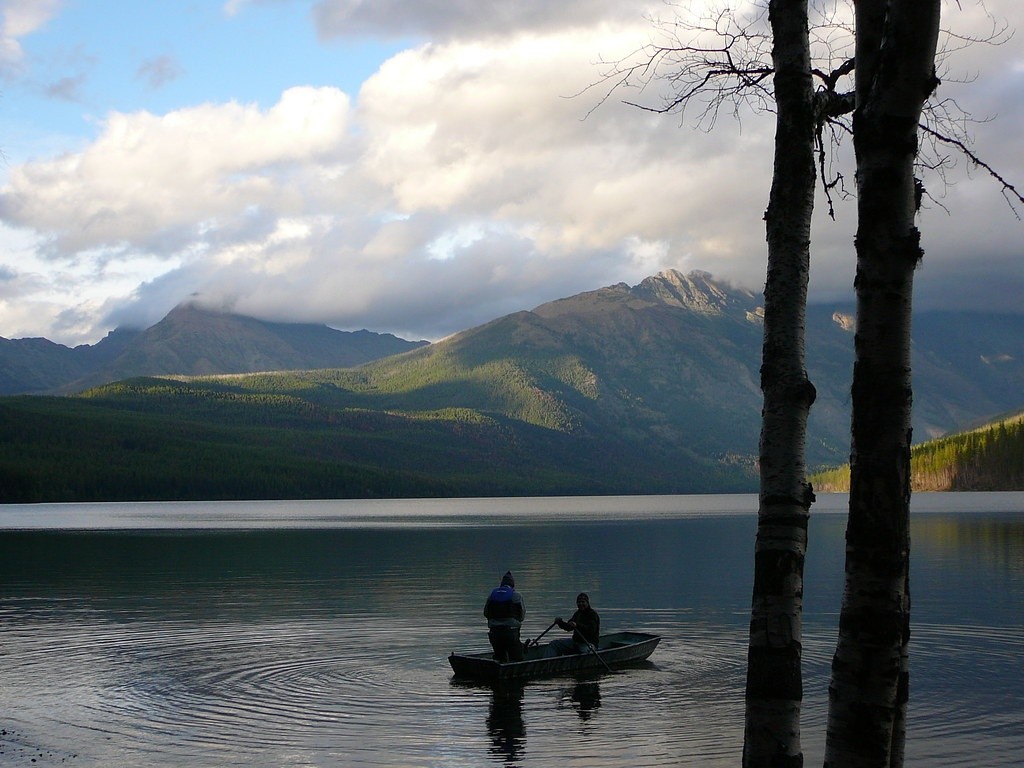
[450,631,661,682]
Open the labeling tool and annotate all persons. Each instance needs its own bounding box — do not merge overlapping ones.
[543,593,600,658]
[484,571,526,663]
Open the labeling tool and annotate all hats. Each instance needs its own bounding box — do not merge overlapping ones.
[500,570,515,589]
[577,593,590,604]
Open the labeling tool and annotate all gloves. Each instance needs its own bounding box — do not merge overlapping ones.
[568,621,576,627]
[555,616,561,624]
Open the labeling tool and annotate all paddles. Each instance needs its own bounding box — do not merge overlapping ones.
[574,628,613,676]
[529,622,556,646]
